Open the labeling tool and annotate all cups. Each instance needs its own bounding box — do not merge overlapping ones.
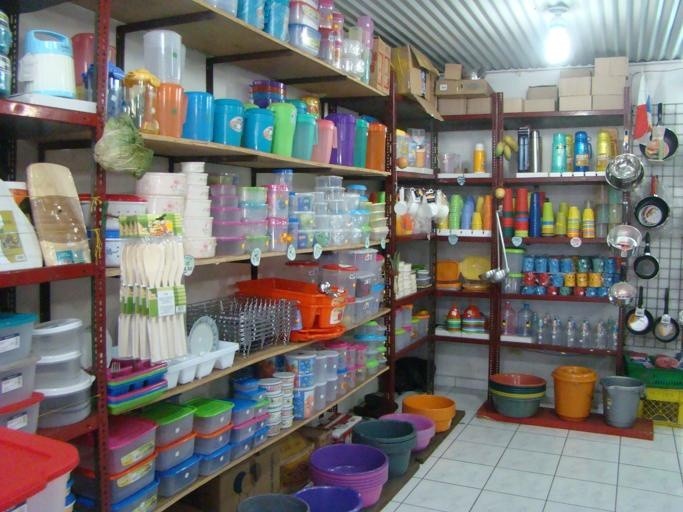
[141,28,184,84]
[439,152,457,174]
[203,1,373,84]
[435,192,494,231]
[522,255,615,299]
[183,90,216,142]
[143,78,189,138]
[241,105,273,153]
[248,78,387,173]
[502,187,595,239]
[212,96,243,147]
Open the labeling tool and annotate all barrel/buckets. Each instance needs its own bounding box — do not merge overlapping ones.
[599,376,646,428]
[549,366,597,423]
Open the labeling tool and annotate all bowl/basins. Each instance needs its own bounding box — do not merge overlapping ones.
[487,373,545,419]
[459,256,493,284]
[234,394,455,511]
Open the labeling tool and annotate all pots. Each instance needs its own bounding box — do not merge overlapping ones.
[606,200,645,258]
[636,174,671,230]
[606,129,646,192]
[642,102,679,162]
[625,286,654,335]
[607,260,636,306]
[636,232,661,278]
[655,283,679,344]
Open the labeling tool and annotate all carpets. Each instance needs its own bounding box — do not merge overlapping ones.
[357,459,420,512]
[477,400,654,442]
[410,409,466,465]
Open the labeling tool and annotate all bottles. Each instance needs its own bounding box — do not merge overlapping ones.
[500,300,617,351]
[81,64,158,134]
[474,142,485,173]
[519,136,528,170]
[552,129,617,174]
[529,130,542,173]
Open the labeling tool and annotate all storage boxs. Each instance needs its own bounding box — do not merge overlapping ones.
[177,443,283,511]
[391,38,630,115]
[275,433,315,495]
[298,422,353,452]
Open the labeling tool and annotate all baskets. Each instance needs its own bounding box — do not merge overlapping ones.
[622,353,683,428]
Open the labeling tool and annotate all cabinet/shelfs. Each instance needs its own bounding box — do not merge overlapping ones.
[431,90,496,414]
[496,85,630,379]
[0,0,104,512]
[324,88,433,422]
[11,1,395,512]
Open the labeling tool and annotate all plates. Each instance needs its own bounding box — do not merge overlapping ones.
[186,316,219,355]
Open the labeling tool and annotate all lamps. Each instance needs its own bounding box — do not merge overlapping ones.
[539,1,572,64]
